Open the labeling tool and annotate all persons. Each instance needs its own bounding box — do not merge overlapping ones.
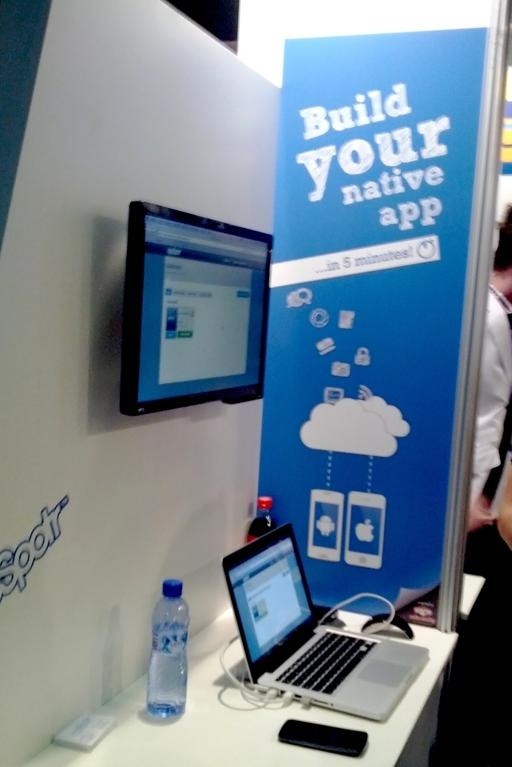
[453,201,511,548]
[425,457,512,767]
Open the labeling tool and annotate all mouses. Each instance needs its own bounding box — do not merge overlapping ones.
[361,613,414,639]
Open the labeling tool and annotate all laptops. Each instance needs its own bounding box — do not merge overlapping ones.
[222,522,430,722]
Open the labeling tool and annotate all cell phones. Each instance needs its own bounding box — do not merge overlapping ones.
[344,490,386,569]
[307,489,345,563]
[277,719,368,758]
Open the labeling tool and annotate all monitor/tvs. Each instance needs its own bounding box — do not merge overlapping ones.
[118,201,273,416]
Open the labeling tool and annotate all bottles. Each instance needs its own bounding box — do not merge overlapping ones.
[145,577,190,719]
[244,496,277,544]
